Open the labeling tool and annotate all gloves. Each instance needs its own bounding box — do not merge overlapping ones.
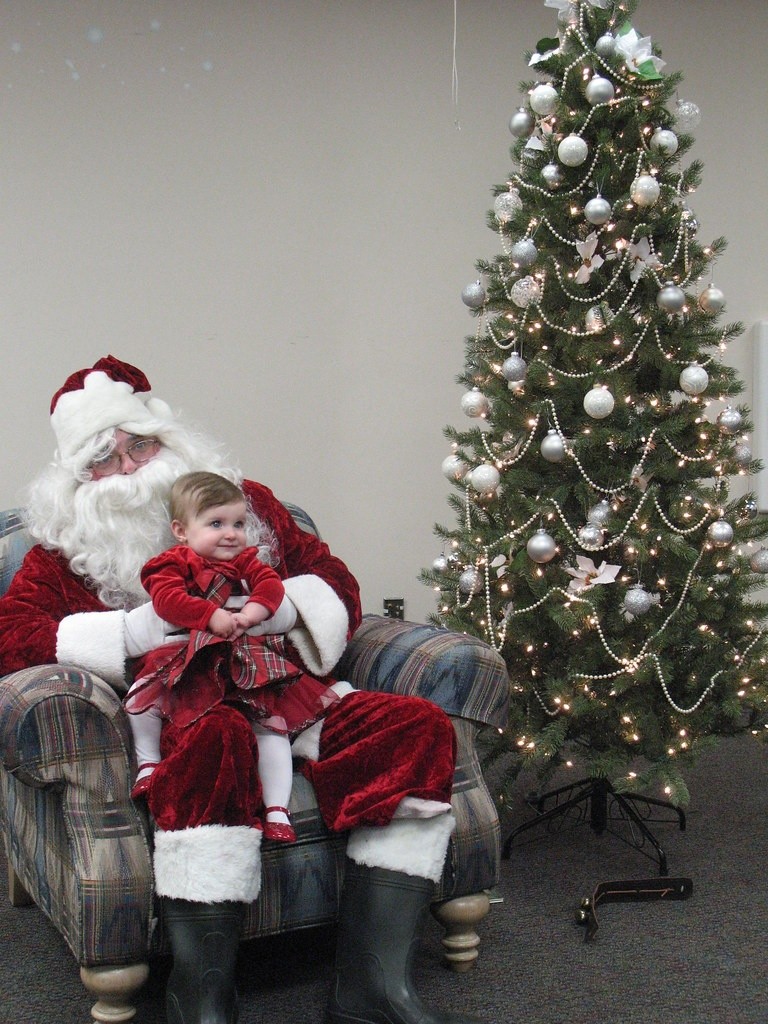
[244,594,297,636]
[123,600,191,658]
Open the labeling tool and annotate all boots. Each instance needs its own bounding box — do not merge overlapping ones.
[153,824,262,1024]
[327,812,456,1024]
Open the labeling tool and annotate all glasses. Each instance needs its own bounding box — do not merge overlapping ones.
[88,438,161,476]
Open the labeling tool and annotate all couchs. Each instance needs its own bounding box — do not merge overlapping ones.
[0,510,512,1024]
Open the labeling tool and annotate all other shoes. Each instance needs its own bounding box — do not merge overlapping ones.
[264,806,297,844]
[132,763,158,799]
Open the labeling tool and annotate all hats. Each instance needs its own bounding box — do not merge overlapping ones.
[50,355,152,458]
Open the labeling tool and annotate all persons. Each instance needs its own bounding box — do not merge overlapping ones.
[124,471,297,842]
[0,354,460,1024]
